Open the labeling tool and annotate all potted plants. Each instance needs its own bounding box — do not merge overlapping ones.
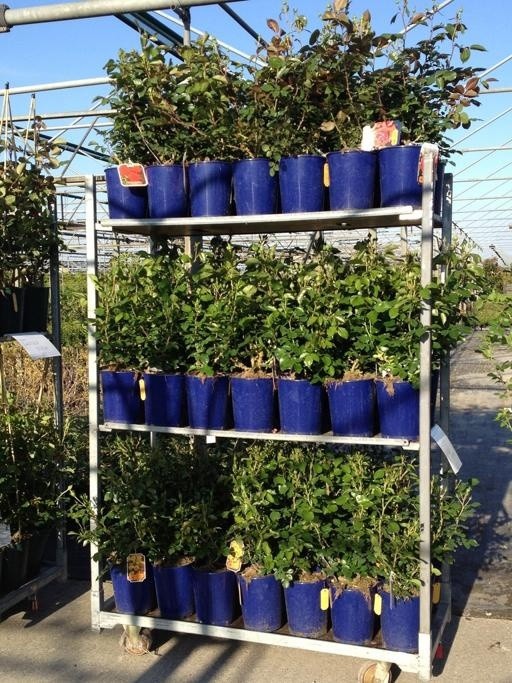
[0,0,512,649]
